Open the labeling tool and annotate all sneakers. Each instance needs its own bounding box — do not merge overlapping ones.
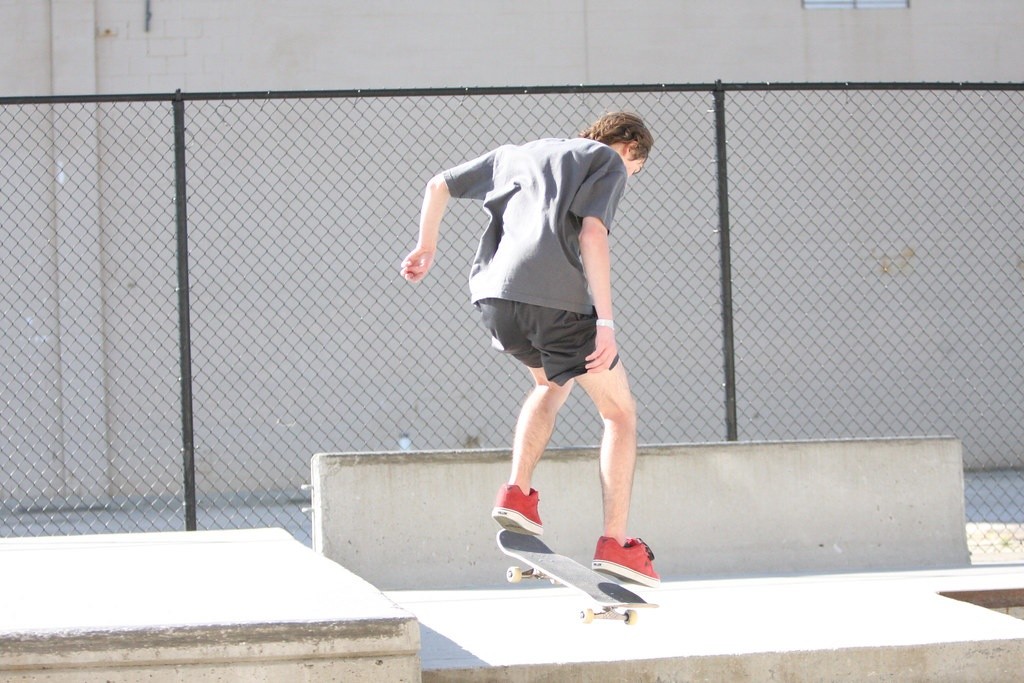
[492,483,544,535]
[592,535,661,589]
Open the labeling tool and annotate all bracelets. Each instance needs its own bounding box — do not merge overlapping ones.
[595,319,615,330]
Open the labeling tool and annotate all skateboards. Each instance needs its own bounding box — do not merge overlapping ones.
[497,527,659,625]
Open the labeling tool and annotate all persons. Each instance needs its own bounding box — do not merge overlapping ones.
[398,112,661,588]
